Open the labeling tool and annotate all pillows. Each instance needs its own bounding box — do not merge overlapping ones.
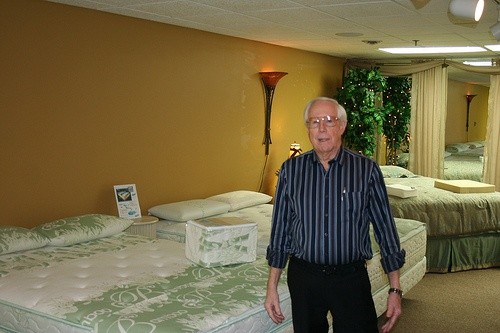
[445,142,480,156]
[149,190,272,221]
[380,166,417,178]
[0,213,133,255]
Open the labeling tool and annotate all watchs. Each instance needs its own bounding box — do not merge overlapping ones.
[388,288,404,298]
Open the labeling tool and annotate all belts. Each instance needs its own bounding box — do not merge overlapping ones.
[293,260,368,273]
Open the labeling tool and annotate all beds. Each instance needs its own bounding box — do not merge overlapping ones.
[0,233,293,333]
[158,204,426,317]
[384,177,500,272]
[446,147,484,178]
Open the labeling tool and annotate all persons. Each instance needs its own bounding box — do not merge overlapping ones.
[263,97,406,333]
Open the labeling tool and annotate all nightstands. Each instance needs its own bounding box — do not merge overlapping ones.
[125,216,159,236]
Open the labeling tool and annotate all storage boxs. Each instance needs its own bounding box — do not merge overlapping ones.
[184,218,258,267]
[435,180,495,193]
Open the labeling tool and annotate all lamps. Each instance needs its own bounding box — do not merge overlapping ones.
[489,22,500,42]
[290,143,301,157]
[466,94,476,132]
[449,0,485,21]
[260,72,289,155]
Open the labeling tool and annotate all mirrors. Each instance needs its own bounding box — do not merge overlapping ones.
[445,65,491,179]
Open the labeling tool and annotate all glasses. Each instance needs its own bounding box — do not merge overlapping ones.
[305,116,343,127]
